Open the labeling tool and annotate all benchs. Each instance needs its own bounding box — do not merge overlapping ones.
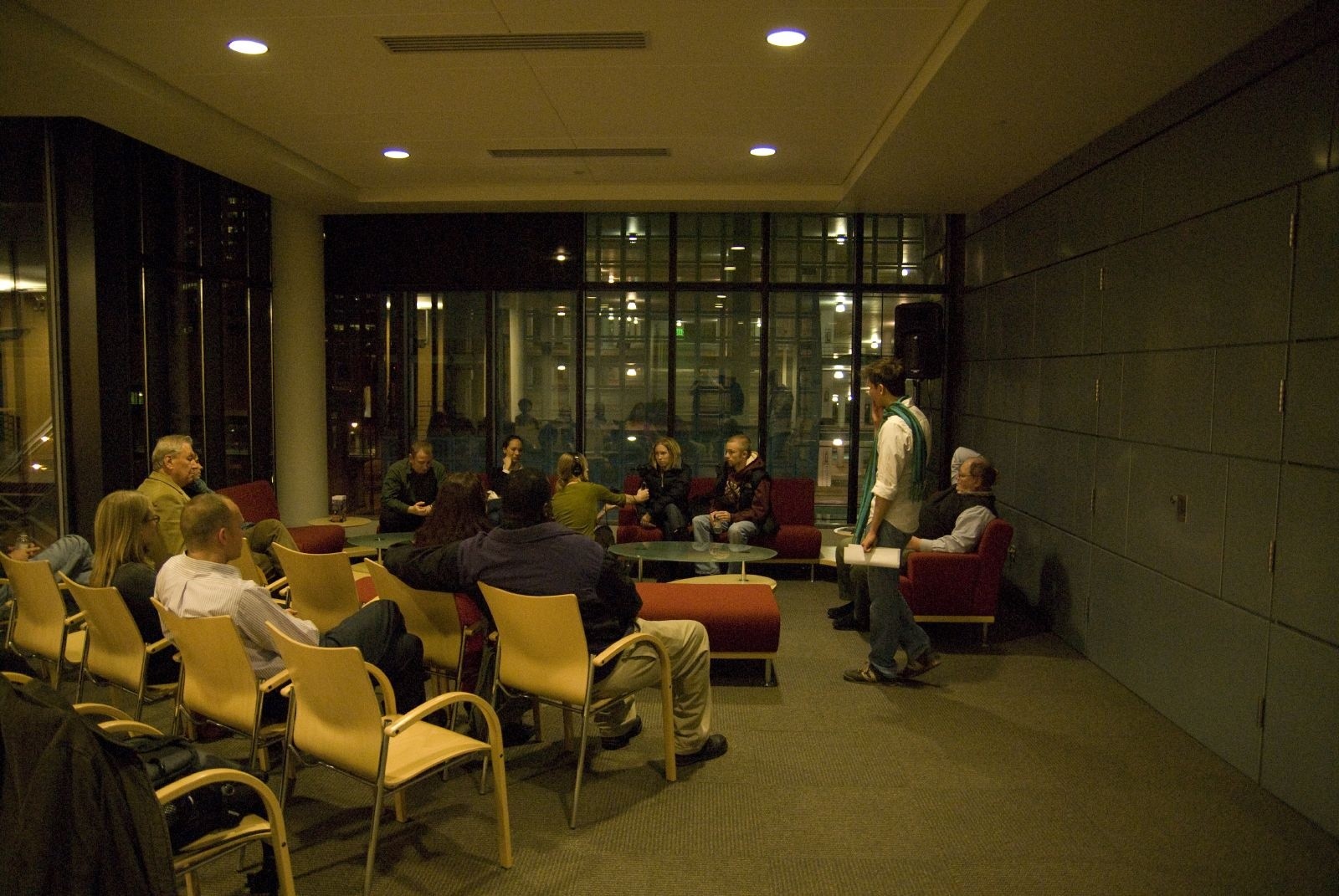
[214,481,346,554]
[633,582,781,687]
[899,519,1014,647]
[617,476,822,582]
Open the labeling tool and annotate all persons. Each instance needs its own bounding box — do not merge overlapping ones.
[549,452,649,574]
[692,434,778,575]
[425,370,793,480]
[153,493,448,729]
[844,355,942,683]
[90,490,182,686]
[636,436,693,583]
[0,535,93,619]
[138,435,300,589]
[490,435,524,500]
[384,468,728,767]
[378,441,448,532]
[412,473,536,741]
[828,447,999,629]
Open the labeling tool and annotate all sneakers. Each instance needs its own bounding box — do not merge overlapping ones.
[844,665,896,684]
[896,651,942,677]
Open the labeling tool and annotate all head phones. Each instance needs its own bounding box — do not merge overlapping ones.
[569,452,583,475]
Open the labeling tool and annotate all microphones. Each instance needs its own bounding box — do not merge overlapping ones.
[642,475,649,490]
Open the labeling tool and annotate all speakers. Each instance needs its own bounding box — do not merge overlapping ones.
[893,301,946,379]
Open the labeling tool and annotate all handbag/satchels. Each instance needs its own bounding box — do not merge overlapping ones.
[119,734,268,851]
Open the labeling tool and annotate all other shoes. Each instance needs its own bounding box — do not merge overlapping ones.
[827,601,855,618]
[601,715,642,749]
[832,616,870,631]
[502,723,537,746]
[675,734,727,767]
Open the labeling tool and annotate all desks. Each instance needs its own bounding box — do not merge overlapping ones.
[309,516,371,528]
[347,532,415,561]
[608,542,778,591]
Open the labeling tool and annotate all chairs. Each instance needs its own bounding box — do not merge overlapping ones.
[1,536,679,895]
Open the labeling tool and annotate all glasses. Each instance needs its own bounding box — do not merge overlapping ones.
[143,515,161,524]
[957,470,978,481]
[16,536,28,544]
